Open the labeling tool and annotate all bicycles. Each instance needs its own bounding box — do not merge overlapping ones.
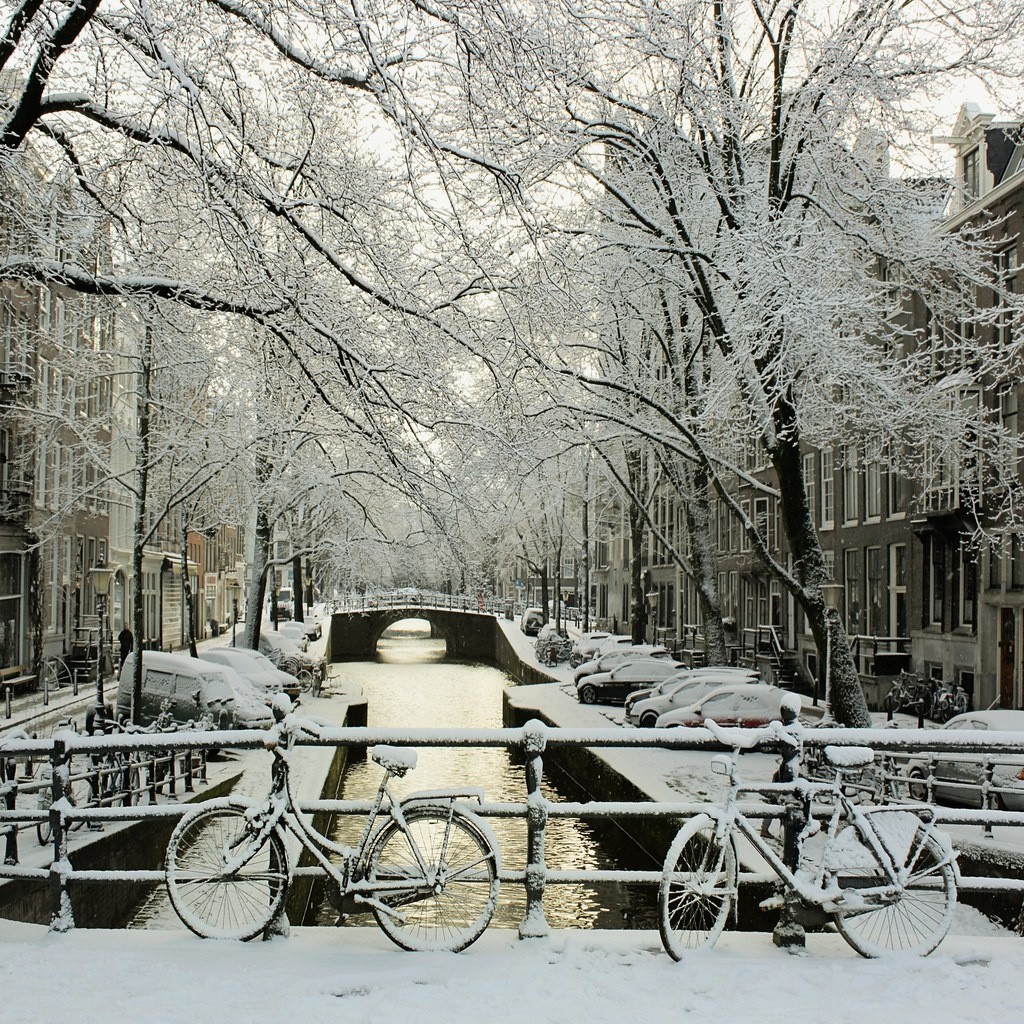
[35,708,206,844]
[657,719,963,962]
[164,693,501,953]
[883,669,968,723]
[261,646,312,693]
[535,634,578,667]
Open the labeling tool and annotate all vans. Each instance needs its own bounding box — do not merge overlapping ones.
[116,650,276,730]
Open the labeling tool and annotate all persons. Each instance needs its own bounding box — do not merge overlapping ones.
[118,622,133,673]
[245,602,248,622]
[760,764,793,839]
[613,616,618,634]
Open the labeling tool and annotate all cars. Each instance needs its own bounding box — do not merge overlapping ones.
[537,624,570,640]
[281,622,308,646]
[573,633,612,650]
[577,659,688,704]
[209,647,302,703]
[228,630,315,671]
[655,684,791,727]
[199,650,283,693]
[520,608,543,636]
[599,636,646,655]
[625,667,767,719]
[278,626,306,652]
[305,616,322,642]
[574,647,673,687]
[906,710,1024,811]
[631,677,759,728]
